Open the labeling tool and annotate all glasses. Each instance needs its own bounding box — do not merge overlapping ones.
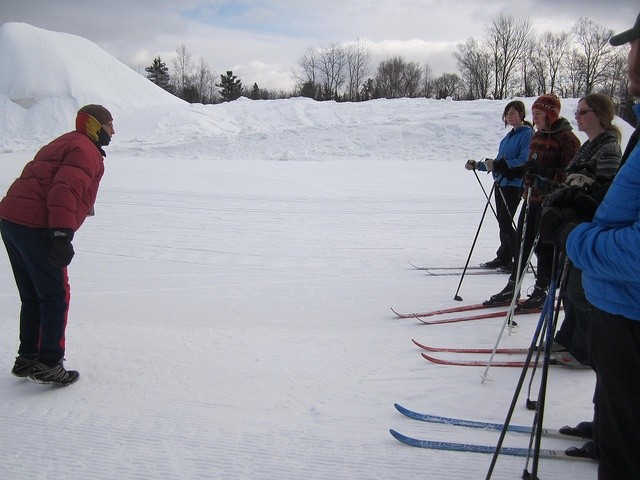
[575,108,596,115]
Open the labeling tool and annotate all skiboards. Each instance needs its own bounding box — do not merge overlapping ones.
[390,403,600,463]
[390,296,564,324]
[408,260,537,276]
[412,338,592,369]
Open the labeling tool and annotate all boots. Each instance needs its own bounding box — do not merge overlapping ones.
[491,279,520,302]
[518,293,546,309]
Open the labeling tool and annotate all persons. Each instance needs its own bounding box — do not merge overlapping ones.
[0,105,115,386]
[557,14,640,480]
[524,93,623,369]
[490,93,580,310]
[465,101,535,273]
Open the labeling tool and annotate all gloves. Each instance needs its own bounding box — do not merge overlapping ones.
[539,200,576,249]
[485,158,493,171]
[465,160,479,170]
[52,229,74,267]
[523,160,542,173]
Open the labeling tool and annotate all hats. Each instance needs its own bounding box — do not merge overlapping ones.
[610,13,639,46]
[78,105,113,126]
[532,94,561,130]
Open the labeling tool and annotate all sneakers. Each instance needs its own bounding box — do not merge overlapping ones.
[27,359,80,386]
[543,339,565,352]
[485,258,504,267]
[11,354,40,377]
[557,352,582,366]
[501,263,515,272]
